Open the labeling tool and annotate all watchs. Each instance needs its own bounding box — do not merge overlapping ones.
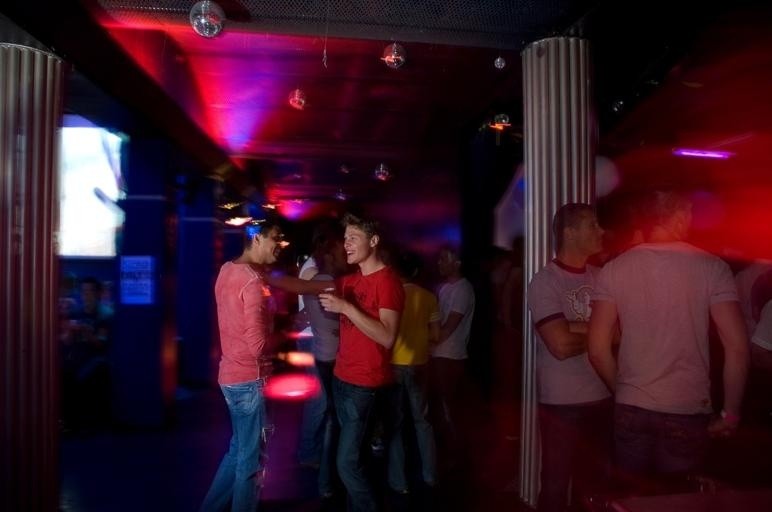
[719,410,742,425]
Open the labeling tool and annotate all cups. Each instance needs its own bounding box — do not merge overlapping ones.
[322,287,335,296]
[302,308,313,328]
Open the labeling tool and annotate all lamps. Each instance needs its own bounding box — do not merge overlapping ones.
[188,0,232,39]
[374,162,391,182]
[380,44,405,68]
[490,113,514,134]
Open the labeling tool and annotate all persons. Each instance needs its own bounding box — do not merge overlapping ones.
[527,203,616,512]
[200,208,310,511]
[305,237,347,497]
[265,216,402,512]
[430,243,474,480]
[386,249,443,493]
[586,186,748,495]
[66,278,117,360]
